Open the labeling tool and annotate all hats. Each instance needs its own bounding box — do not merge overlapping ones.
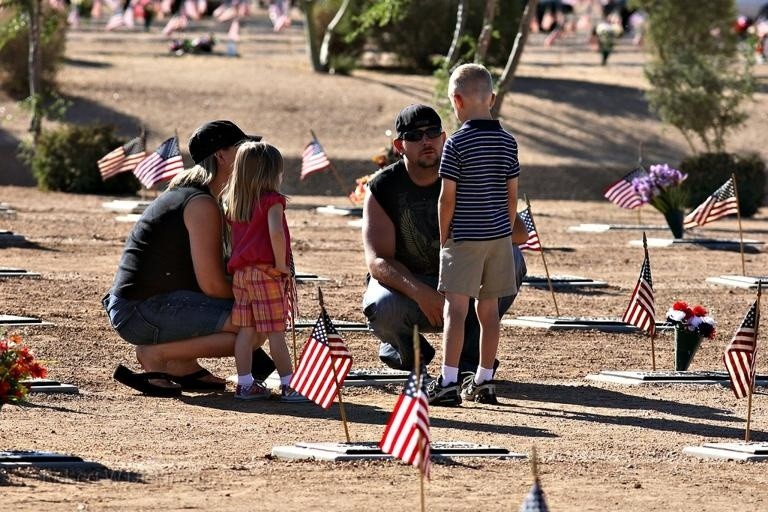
[188,119,263,165]
[395,103,442,138]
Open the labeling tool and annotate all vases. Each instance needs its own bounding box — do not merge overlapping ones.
[657,208,688,241]
[671,327,704,371]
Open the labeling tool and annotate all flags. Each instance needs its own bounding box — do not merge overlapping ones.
[603,166,650,209]
[683,177,738,230]
[379,353,431,481]
[722,297,760,400]
[48,0,305,43]
[517,207,541,252]
[288,306,353,410]
[528,0,644,51]
[521,481,548,512]
[621,255,656,338]
[287,248,300,326]
[97,133,147,181]
[132,135,185,190]
[300,140,330,181]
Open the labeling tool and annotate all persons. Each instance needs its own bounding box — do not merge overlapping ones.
[422,63,521,407]
[101,120,268,397]
[222,140,315,404]
[362,102,529,380]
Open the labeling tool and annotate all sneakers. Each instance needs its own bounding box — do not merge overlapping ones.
[421,373,463,406]
[459,372,499,406]
[234,379,271,399]
[280,383,309,400]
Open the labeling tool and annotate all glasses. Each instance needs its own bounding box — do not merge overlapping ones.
[403,125,443,142]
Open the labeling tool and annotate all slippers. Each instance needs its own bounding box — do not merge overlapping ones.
[113,364,184,398]
[168,366,228,392]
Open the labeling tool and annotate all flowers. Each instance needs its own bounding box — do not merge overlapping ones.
[627,158,692,215]
[0,331,49,412]
[657,301,718,341]
